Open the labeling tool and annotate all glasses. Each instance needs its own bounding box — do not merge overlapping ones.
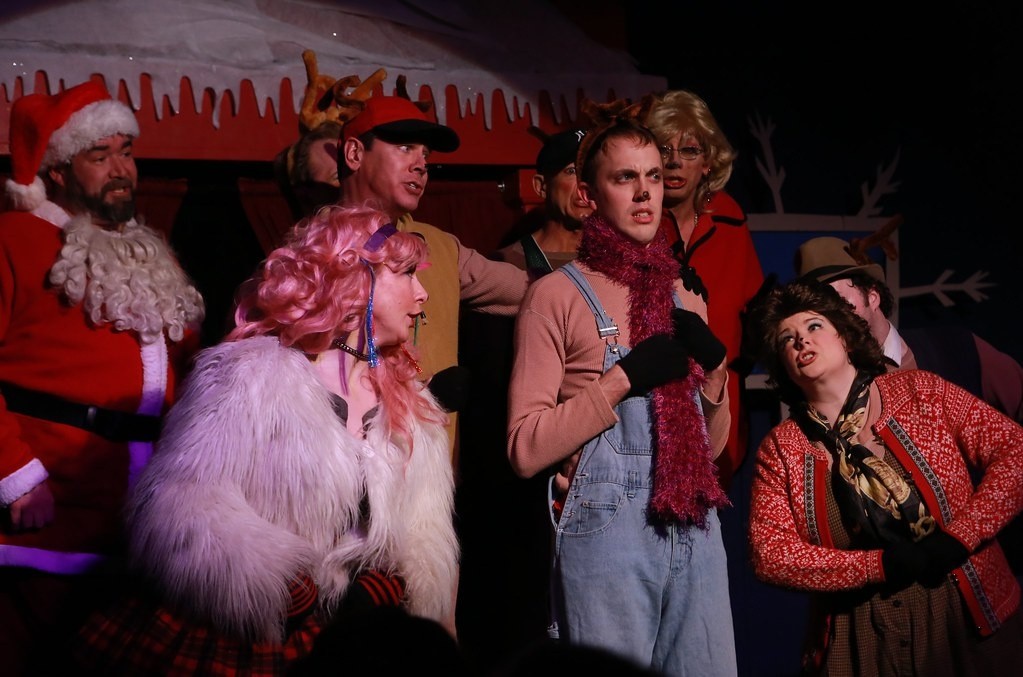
[657,143,704,161]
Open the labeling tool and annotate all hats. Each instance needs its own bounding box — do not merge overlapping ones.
[5,78,140,212]
[793,236,886,290]
[336,96,460,183]
[536,130,588,183]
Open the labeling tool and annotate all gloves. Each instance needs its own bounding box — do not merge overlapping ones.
[615,333,689,397]
[884,531,967,581]
[670,307,728,373]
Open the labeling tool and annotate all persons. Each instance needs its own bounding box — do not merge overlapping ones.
[70,59,1022,676]
[0,78,207,676]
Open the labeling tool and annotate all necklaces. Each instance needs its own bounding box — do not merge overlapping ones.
[334,339,378,362]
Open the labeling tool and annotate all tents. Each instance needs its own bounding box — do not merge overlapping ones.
[0,0,672,263]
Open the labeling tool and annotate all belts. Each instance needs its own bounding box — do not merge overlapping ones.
[1,380,165,444]
